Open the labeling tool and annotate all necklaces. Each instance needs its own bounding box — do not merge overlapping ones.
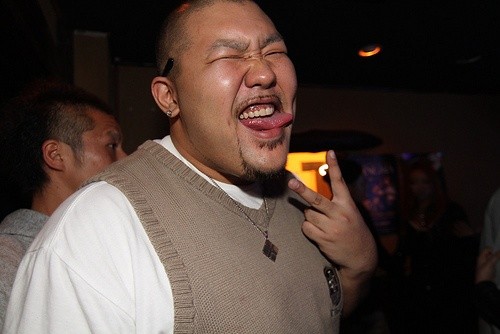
[178,151,279,263]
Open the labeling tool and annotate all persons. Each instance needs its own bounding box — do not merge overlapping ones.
[3,0,378,334]
[338,153,500,334]
[0,84,128,334]
